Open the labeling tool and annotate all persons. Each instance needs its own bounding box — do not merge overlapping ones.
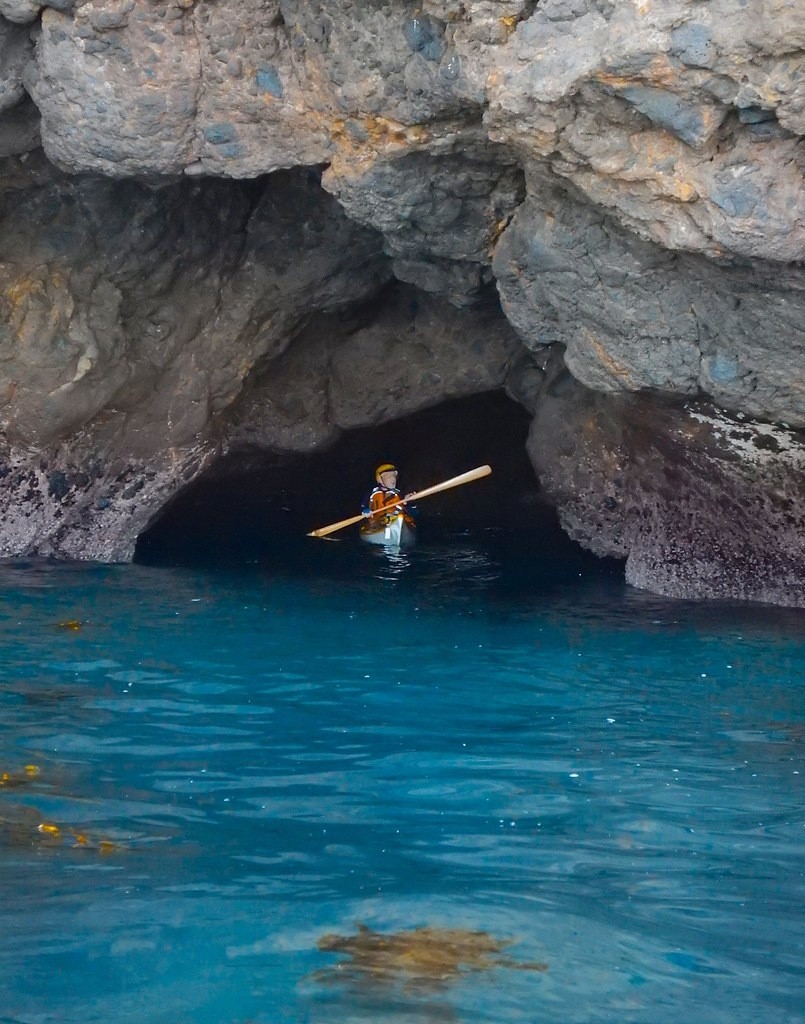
[363,464,419,524]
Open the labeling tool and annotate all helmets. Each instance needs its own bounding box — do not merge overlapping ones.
[375,464,398,479]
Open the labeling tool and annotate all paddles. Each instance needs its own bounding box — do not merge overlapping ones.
[308,464,492,538]
